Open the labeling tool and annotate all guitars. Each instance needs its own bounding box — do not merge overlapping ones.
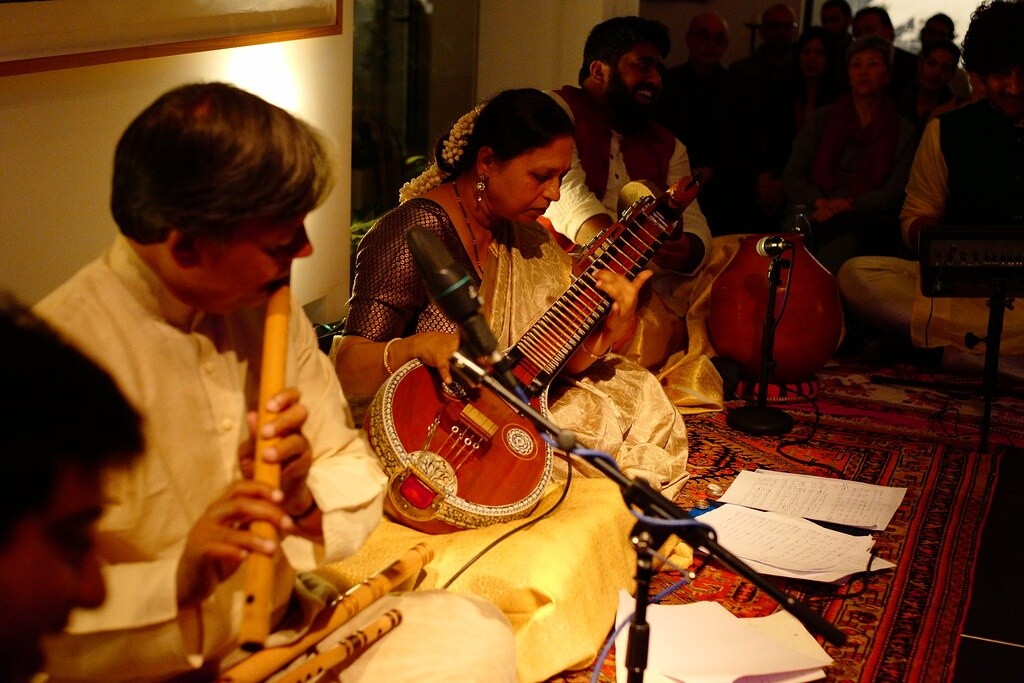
[370,171,702,529]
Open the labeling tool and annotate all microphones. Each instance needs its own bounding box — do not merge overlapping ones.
[406,225,531,410]
[756,237,794,258]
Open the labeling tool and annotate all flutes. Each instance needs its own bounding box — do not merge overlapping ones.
[219,542,435,683]
[237,280,292,653]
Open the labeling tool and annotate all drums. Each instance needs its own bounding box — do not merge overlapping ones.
[616,179,683,239]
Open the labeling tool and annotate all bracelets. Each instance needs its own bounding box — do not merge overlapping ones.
[580,342,614,362]
[381,336,400,375]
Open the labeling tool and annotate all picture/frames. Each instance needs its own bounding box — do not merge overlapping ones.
[0,0,343,78]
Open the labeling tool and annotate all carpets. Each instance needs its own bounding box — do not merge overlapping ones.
[546,404,1004,683]
[736,324,1024,450]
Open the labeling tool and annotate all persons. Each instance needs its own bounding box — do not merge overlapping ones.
[28,83,393,678]
[336,88,687,503]
[544,0,1024,368]
[0,288,148,683]
